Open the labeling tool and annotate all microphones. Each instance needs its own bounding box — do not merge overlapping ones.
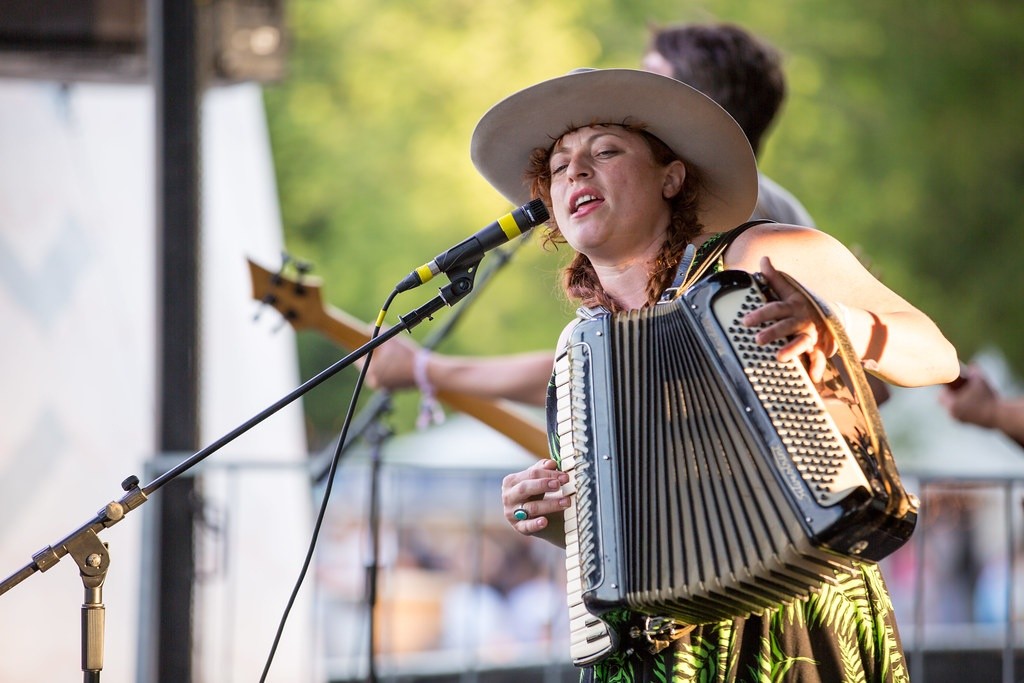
[494,226,536,264]
[396,199,550,292]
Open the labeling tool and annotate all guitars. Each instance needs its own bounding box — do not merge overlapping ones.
[246,244,551,461]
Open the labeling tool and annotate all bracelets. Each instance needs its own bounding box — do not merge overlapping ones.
[413,348,443,428]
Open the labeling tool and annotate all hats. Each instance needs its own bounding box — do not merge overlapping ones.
[469,67,758,251]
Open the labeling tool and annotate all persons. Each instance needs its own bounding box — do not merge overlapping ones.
[348,25,890,409]
[465,69,1024,683]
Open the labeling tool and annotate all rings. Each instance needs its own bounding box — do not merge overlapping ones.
[512,503,529,522]
